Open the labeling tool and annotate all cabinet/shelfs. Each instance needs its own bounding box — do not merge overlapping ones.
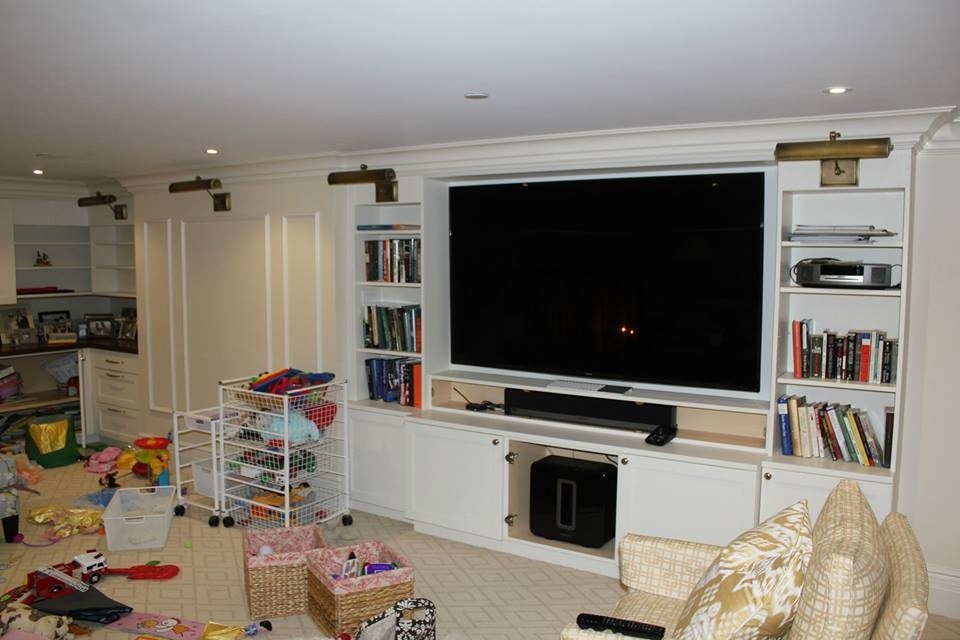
[13,224,137,298]
[348,186,913,579]
[0,348,147,449]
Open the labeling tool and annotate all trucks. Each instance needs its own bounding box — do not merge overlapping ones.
[26,548,109,598]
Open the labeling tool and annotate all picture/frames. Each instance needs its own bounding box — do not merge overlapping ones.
[118,320,137,341]
[38,310,72,342]
[84,313,116,339]
[0,304,35,345]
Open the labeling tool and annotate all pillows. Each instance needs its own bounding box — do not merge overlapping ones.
[670,476,890,640]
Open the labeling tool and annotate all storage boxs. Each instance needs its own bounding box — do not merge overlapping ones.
[100,486,176,552]
[242,525,415,640]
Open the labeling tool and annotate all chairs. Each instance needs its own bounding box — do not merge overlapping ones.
[562,513,929,640]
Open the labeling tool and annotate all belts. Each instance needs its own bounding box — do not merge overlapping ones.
[308,540,414,640]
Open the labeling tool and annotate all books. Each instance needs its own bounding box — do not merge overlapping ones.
[365,239,421,284]
[778,394,894,468]
[788,225,889,244]
[358,224,421,230]
[363,302,421,354]
[365,357,421,408]
[792,319,897,385]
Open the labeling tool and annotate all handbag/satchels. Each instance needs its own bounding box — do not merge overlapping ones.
[353,598,437,640]
[25,415,81,469]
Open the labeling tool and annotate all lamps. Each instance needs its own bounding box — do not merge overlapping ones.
[169,176,231,212]
[774,132,894,186]
[78,192,127,220]
[328,164,398,202]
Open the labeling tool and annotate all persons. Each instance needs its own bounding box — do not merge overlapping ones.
[5,311,30,340]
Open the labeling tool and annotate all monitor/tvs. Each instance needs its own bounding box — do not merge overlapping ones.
[450,170,776,401]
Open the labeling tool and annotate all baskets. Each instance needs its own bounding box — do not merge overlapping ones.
[241,524,328,621]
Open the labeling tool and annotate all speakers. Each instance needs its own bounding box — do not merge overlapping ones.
[529,456,617,548]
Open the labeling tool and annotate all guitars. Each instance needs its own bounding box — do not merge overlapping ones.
[108,564,180,580]
